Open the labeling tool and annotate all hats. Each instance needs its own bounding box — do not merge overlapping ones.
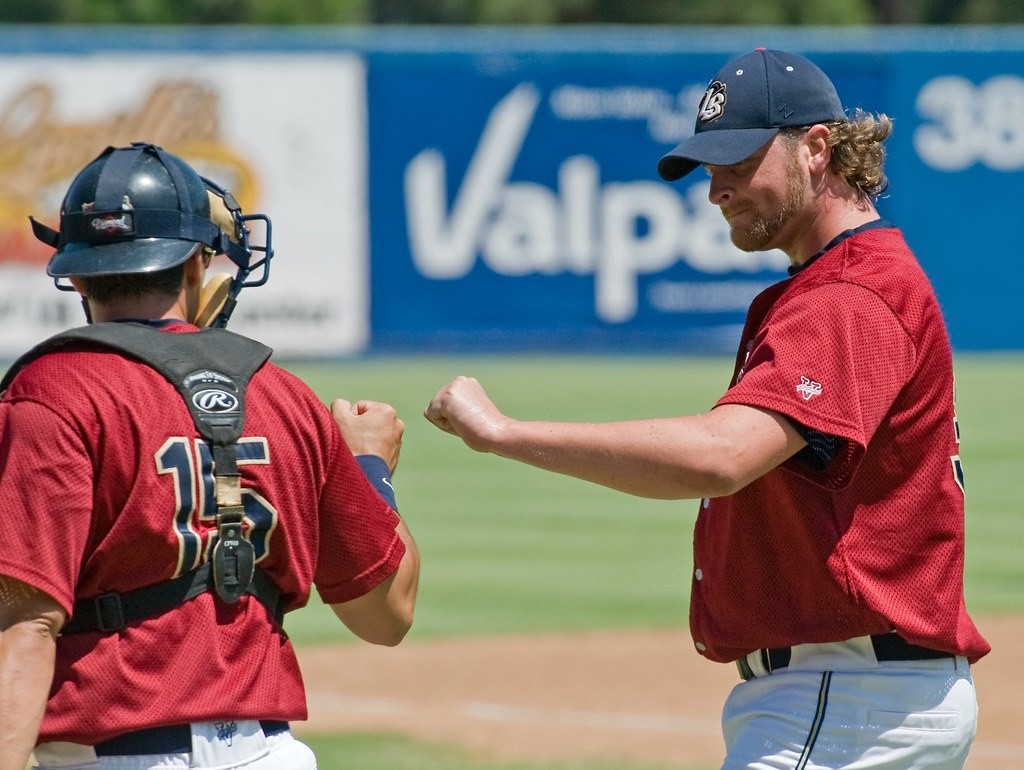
[658,48,849,181]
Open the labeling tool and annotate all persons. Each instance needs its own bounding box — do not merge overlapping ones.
[0,142,422,770]
[424,48,990,770]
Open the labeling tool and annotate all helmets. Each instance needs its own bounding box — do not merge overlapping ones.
[47,141,211,277]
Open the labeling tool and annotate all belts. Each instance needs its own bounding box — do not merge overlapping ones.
[736,633,955,681]
[94,719,290,757]
